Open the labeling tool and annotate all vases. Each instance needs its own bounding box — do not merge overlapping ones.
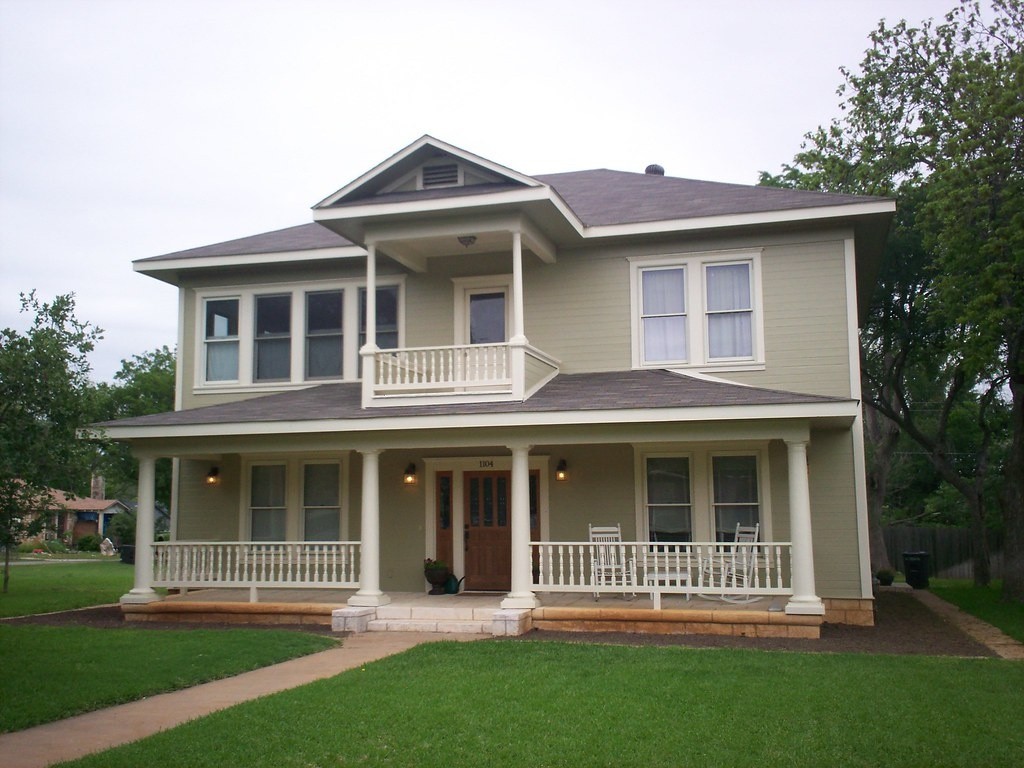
[424,568,450,595]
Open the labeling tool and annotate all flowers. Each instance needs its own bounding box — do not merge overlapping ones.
[424,558,448,568]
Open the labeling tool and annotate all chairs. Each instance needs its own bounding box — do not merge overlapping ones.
[589,522,636,597]
[698,522,765,604]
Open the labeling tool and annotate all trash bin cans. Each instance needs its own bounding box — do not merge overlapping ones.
[901,551,931,590]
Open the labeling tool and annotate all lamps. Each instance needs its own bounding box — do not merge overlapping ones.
[404,463,417,485]
[206,467,219,483]
[556,459,568,481]
[458,237,477,248]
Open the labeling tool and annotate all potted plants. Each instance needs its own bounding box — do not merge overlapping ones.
[873,566,897,586]
[108,508,137,564]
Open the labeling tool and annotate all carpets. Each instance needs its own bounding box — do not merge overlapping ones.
[456,593,507,596]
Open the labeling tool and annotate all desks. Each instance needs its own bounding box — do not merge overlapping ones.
[647,571,694,600]
